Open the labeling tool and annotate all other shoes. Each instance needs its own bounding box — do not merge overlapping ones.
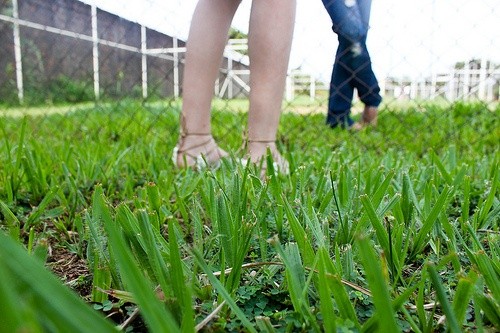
[236,155,290,179]
[172,145,232,169]
[362,106,377,127]
[350,122,361,134]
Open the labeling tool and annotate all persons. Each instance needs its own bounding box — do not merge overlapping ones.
[172,0,293,178]
[322,0,383,134]
